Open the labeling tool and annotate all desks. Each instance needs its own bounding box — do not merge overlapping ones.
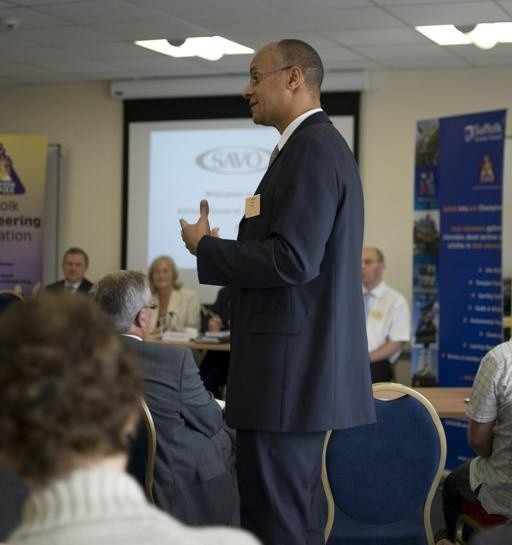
[145,333,230,351]
[410,388,474,418]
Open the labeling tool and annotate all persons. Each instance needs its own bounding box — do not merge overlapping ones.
[201,287,231,334]
[441,336,512,544]
[42,247,93,294]
[144,255,198,331]
[89,269,240,527]
[0,294,260,543]
[179,40,377,545]
[362,243,411,383]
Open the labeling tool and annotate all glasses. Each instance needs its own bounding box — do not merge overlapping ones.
[149,302,159,310]
[251,64,302,83]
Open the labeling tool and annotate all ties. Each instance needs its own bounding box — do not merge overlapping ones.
[65,285,73,292]
[269,145,278,167]
[363,291,372,318]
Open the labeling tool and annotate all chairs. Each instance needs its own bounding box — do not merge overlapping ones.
[319,383,446,544]
[126,393,156,508]
